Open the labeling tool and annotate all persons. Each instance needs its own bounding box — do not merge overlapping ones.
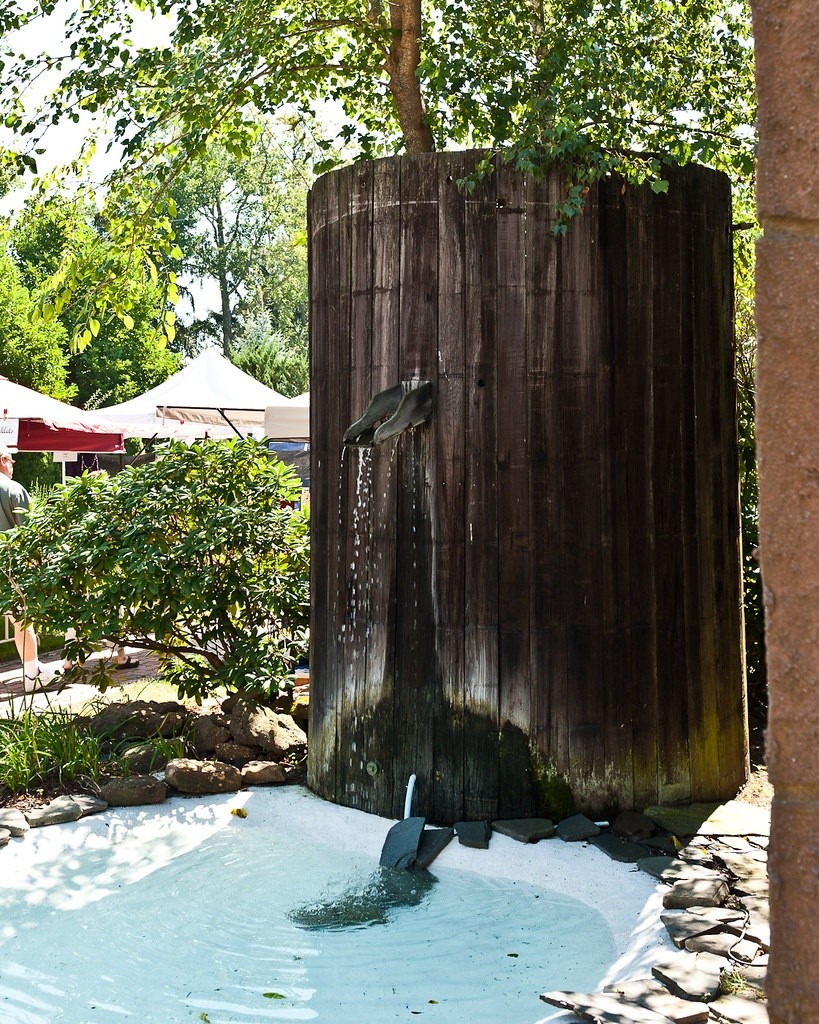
[0,443,65,693]
[63,471,139,672]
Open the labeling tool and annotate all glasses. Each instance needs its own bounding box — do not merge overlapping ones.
[4,457,16,466]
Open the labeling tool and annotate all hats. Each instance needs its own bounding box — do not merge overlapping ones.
[0,442,18,456]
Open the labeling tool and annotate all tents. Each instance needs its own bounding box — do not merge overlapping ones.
[88,348,290,467]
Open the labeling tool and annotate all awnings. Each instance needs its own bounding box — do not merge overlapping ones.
[0,375,127,471]
[264,392,310,454]
